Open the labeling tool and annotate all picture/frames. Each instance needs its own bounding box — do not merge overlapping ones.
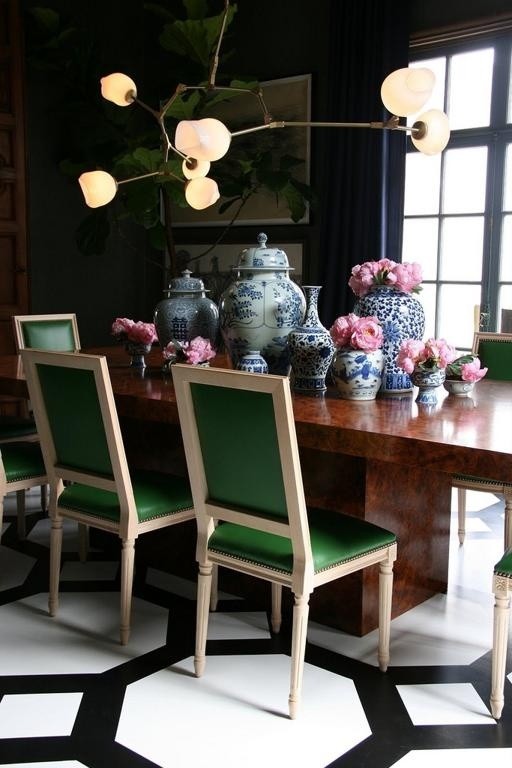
[159,74,313,226]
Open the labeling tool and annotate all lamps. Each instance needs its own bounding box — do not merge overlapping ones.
[78,0,451,210]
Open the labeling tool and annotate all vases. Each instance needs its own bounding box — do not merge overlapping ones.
[408,366,447,404]
[286,286,335,395]
[330,348,385,401]
[123,343,153,369]
[236,350,269,372]
[353,284,426,394]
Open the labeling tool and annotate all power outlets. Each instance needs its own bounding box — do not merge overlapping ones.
[171,244,306,278]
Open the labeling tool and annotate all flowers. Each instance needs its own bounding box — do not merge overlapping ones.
[394,338,455,375]
[446,353,489,383]
[329,313,385,354]
[348,257,424,298]
[109,317,159,346]
[162,337,215,366]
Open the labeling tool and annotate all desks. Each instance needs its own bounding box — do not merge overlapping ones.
[0,346,512,638]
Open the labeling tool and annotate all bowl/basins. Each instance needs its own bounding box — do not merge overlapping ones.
[441,396,474,410]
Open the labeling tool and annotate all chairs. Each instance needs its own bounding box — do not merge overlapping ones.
[13,314,85,358]
[17,347,194,647]
[457,331,512,557]
[489,555,512,719]
[0,426,55,543]
[169,361,402,722]
